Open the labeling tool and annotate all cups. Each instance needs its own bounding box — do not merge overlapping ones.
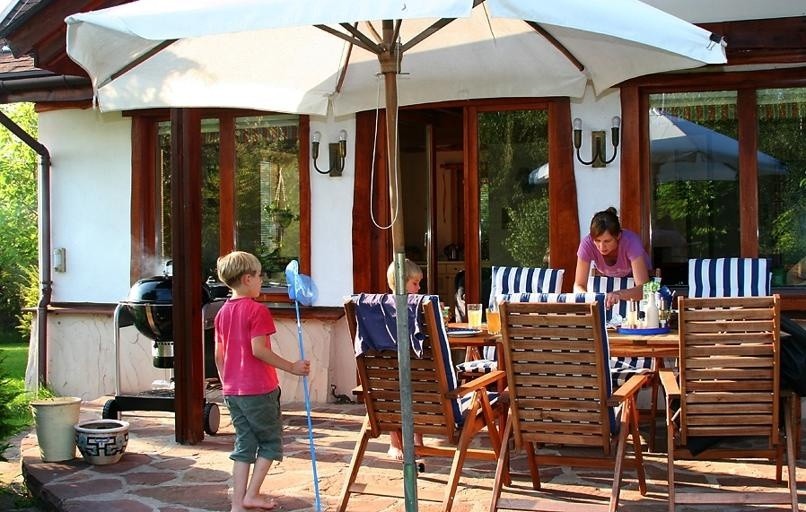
[485,307,501,335]
[467,304,482,328]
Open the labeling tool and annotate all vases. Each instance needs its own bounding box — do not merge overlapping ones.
[73,418,130,465]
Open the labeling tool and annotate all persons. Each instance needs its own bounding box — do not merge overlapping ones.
[387,258,425,460]
[212,249,309,512]
[573,207,652,310]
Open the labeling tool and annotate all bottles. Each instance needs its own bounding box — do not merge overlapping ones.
[643,292,661,329]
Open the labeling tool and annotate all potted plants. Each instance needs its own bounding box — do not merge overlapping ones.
[29,383,82,463]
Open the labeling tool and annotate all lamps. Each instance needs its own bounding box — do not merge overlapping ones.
[311,130,347,176]
[573,116,621,168]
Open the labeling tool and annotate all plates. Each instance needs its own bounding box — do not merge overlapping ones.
[446,331,480,338]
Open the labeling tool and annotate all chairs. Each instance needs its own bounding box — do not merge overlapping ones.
[588,266,661,413]
[455,265,566,385]
[657,293,800,510]
[489,294,648,511]
[686,256,774,313]
[335,292,511,510]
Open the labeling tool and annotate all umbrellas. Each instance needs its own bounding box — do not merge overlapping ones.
[64,0,728,507]
[524,105,787,191]
